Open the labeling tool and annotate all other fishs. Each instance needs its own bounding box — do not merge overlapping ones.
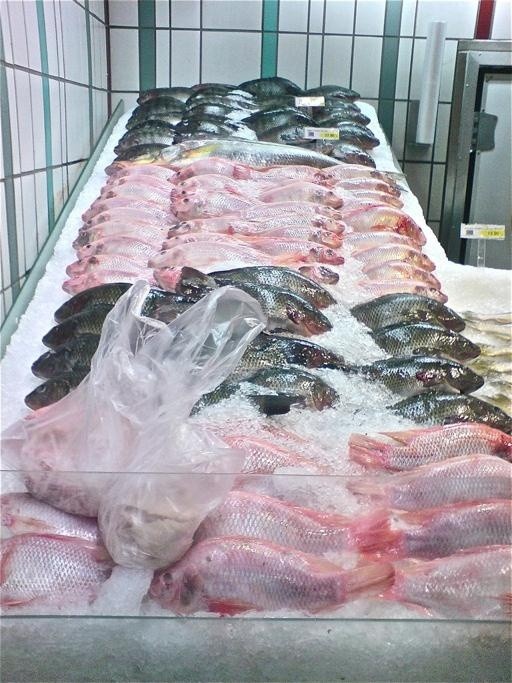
[25,266,512,432]
[0,421,512,616]
[62,157,448,305]
